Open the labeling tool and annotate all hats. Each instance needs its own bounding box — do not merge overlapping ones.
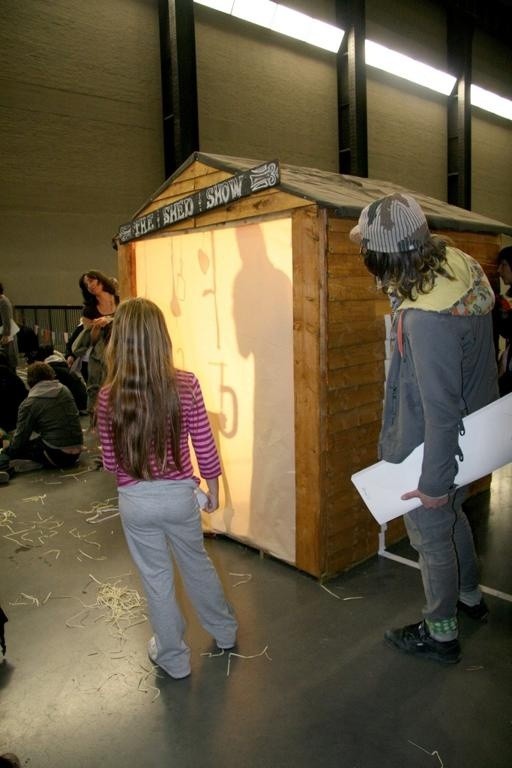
[349,191,431,254]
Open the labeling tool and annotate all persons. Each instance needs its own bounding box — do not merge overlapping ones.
[0,269,120,484]
[492,245,512,394]
[94,297,240,681]
[348,192,501,666]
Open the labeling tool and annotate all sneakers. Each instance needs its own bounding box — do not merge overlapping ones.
[383,618,463,664]
[7,459,42,473]
[456,596,489,623]
[147,635,191,679]
[0,472,9,483]
[216,639,236,649]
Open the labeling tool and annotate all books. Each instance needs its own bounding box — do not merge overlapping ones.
[350,391,512,525]
